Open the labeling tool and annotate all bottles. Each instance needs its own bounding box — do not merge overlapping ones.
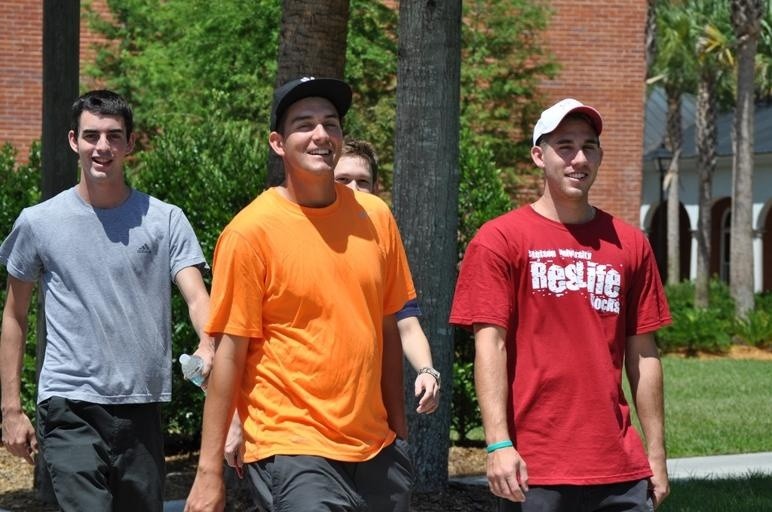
[180,354,205,387]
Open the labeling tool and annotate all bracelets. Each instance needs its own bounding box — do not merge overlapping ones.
[416,366,442,389]
[486,439,513,454]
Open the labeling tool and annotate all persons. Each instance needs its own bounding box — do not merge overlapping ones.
[115,378,116,382]
[183,74,443,512]
[332,137,380,194]
[448,95,672,512]
[0,88,243,512]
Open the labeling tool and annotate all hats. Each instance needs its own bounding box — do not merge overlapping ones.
[533,99,603,147]
[271,76,352,133]
